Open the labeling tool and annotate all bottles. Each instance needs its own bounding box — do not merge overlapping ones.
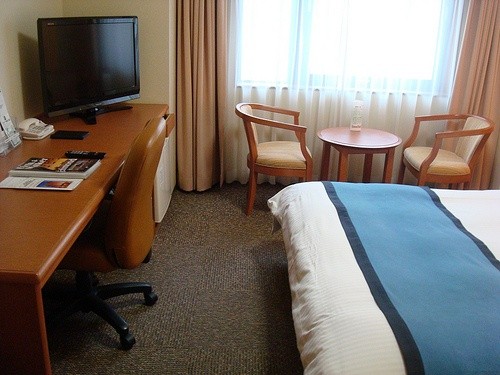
[351,101,362,131]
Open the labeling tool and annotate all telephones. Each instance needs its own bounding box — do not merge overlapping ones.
[15,117,55,141]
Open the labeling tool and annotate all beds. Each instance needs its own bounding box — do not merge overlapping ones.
[266,181,500,375]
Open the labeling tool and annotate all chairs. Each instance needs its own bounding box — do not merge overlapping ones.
[55,117,167,350]
[235,103,314,215]
[396,114,496,190]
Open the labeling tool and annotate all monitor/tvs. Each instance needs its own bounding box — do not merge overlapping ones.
[37,16,141,125]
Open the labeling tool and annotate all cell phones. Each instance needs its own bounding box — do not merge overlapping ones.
[50,130,90,140]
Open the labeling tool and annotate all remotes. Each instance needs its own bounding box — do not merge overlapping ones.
[65,151,106,159]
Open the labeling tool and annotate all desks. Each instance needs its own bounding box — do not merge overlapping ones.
[317,127,403,183]
[1,102,175,375]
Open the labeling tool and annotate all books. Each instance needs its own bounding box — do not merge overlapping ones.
[9,156,102,179]
